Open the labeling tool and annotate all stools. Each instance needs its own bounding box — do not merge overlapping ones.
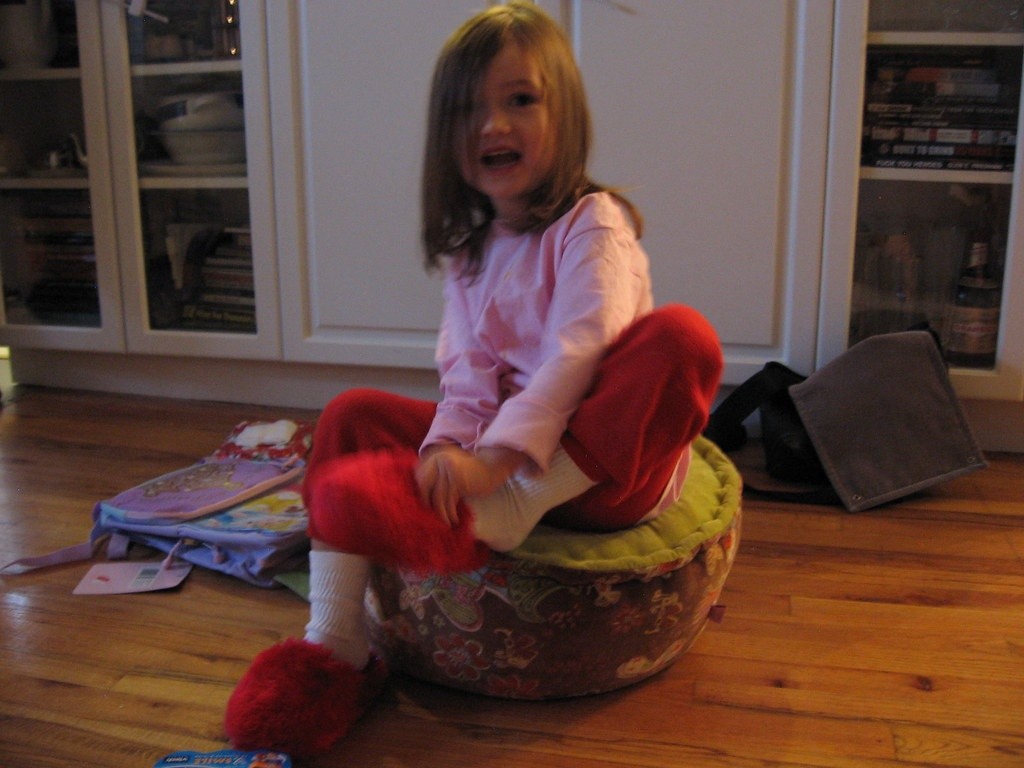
[367,433,742,704]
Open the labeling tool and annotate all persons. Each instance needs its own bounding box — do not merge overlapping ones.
[223,1,724,761]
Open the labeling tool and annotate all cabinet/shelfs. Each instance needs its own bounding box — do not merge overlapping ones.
[1,0,1024,452]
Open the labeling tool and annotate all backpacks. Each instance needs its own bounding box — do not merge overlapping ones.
[0,447,307,588]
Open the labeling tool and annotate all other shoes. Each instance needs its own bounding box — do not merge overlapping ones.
[222,635,388,754]
[310,449,490,576]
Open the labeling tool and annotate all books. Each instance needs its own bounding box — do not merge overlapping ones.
[14,197,100,313]
[182,224,258,333]
[862,48,1016,172]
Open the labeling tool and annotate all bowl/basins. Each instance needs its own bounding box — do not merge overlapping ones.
[147,89,248,166]
